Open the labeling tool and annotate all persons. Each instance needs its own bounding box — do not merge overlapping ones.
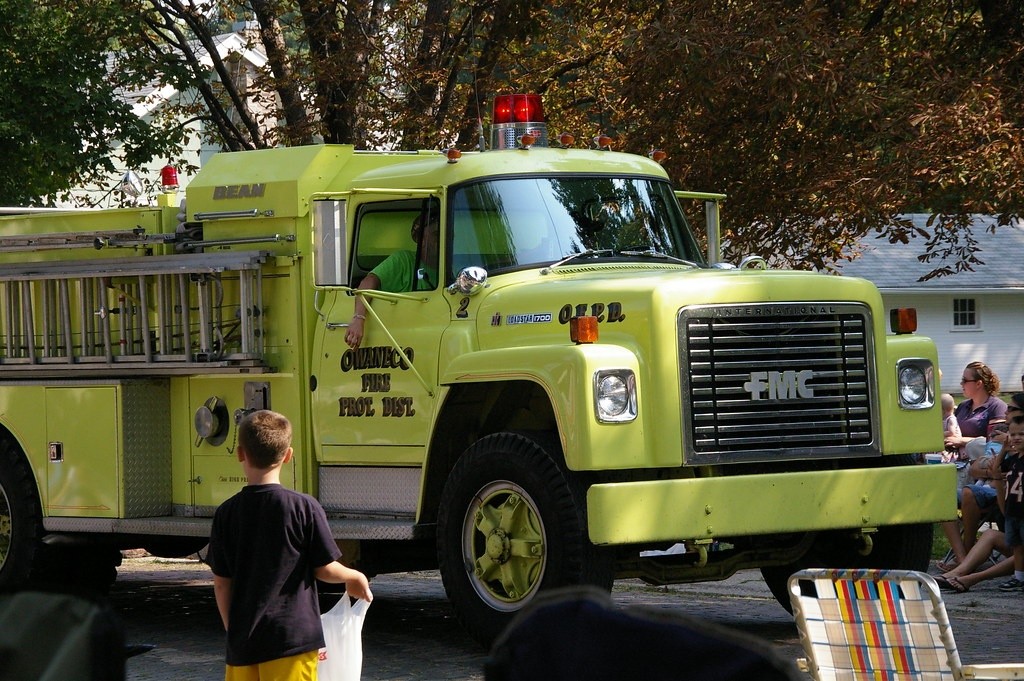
[207,410,371,681]
[344,212,466,352]
[930,362,1024,591]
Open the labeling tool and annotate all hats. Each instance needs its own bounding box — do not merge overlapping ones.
[411,209,443,243]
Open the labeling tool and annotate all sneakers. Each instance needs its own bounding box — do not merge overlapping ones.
[998,575,1024,591]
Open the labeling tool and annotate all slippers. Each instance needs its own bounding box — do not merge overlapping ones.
[936,562,957,572]
[932,575,956,590]
[945,577,970,593]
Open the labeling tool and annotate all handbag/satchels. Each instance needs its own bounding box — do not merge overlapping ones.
[317,591,372,681]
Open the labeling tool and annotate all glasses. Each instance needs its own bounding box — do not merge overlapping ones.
[962,378,978,383]
[1008,406,1024,413]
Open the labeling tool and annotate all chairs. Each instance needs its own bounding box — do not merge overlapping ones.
[788,568,1024,681]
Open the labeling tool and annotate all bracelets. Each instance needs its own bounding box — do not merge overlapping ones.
[353,314,366,320]
[986,469,989,478]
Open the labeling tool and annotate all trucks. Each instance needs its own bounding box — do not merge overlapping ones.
[0,92,959,668]
